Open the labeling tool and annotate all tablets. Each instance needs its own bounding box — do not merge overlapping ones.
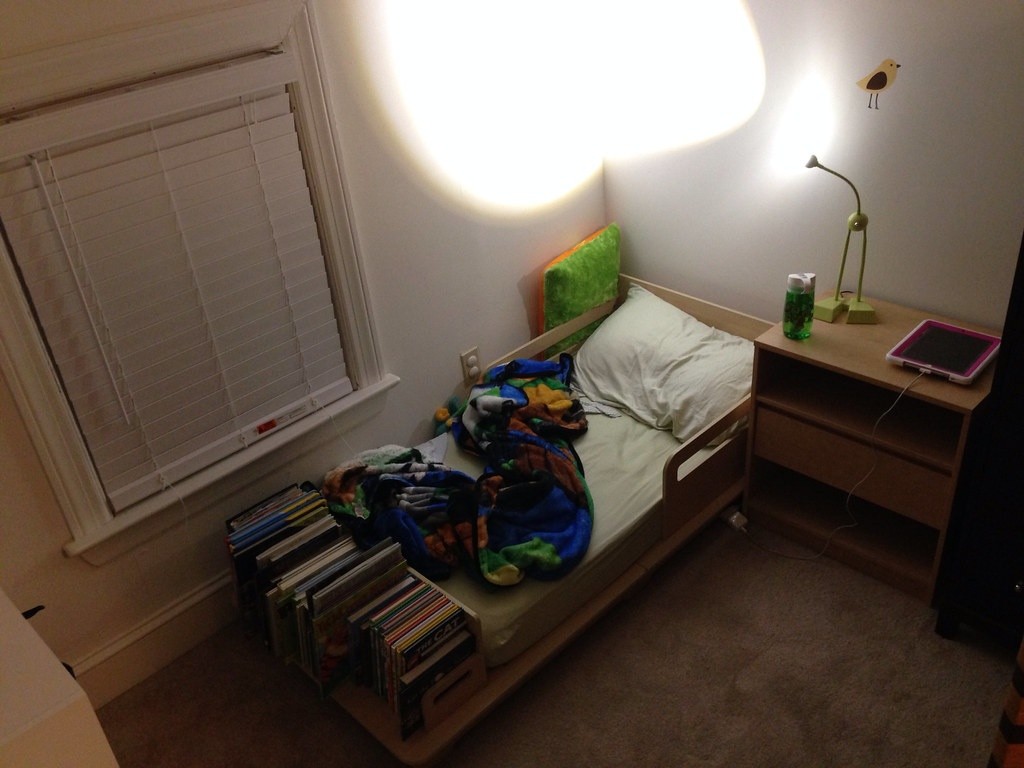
[884,318,1003,385]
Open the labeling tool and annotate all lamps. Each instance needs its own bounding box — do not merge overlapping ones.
[805,155,877,323]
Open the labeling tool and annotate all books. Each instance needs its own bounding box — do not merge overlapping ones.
[222,484,487,738]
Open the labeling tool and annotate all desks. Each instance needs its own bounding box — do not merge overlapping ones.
[0,586,120,768]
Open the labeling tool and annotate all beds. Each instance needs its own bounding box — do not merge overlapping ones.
[281,273,778,768]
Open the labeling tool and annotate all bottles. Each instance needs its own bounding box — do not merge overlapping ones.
[782,272,816,341]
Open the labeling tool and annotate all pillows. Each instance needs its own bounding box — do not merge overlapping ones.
[538,220,620,362]
[570,282,758,449]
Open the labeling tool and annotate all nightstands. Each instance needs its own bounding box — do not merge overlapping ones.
[738,289,1008,604]
